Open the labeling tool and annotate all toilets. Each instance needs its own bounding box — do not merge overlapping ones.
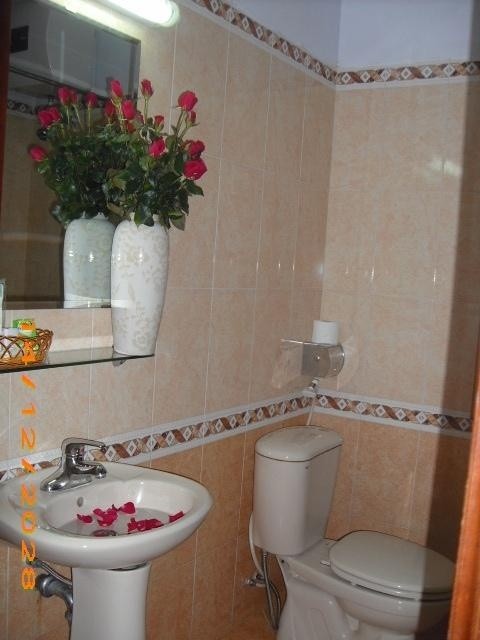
[251,424,456,640]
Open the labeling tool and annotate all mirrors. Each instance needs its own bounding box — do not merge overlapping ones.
[0,0,143,310]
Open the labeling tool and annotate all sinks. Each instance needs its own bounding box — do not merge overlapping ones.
[0,460,215,570]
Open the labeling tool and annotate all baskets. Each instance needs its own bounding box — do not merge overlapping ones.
[0,328,54,370]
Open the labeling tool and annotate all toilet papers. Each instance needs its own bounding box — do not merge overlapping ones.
[311,319,340,346]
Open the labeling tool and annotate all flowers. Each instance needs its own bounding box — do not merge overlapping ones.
[30,82,103,225]
[101,67,207,231]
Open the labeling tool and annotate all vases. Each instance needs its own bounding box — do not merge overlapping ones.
[109,209,171,359]
[61,209,116,309]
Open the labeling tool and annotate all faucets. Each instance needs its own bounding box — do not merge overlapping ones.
[39,436,108,493]
